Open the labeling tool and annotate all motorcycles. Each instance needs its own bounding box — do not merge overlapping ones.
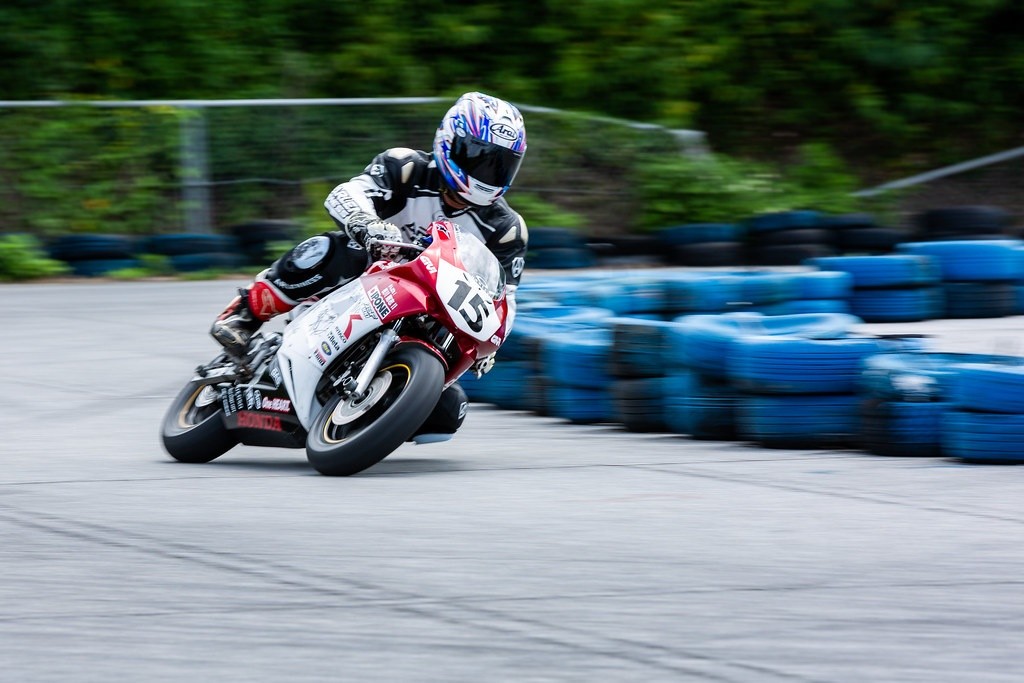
[160,218,511,475]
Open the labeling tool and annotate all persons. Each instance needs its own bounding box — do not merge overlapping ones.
[210,91,529,446]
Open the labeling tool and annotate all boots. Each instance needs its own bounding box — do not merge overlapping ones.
[210,269,301,354]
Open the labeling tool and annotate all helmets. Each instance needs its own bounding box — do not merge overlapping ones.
[433,91,528,205]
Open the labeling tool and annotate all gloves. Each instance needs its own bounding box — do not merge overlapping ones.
[362,221,403,261]
[473,352,497,380]
